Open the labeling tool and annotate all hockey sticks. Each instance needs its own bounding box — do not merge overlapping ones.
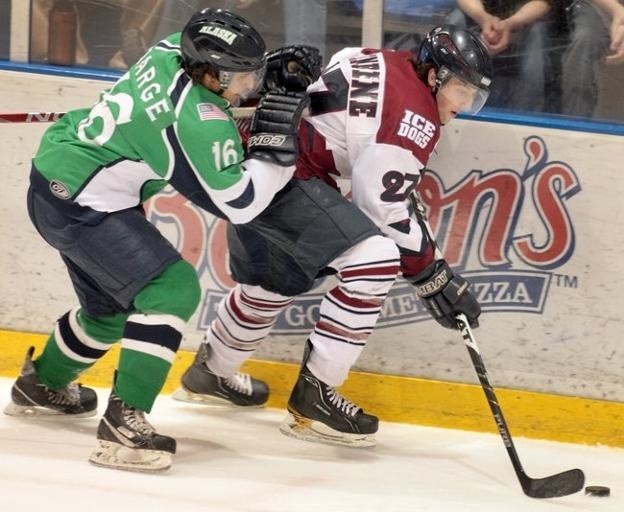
[409,190,584,497]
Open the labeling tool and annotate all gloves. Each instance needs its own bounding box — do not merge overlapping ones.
[263,44,322,91]
[246,87,311,167]
[405,261,480,332]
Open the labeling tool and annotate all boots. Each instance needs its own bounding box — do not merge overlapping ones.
[10,346,98,415]
[287,339,380,434]
[180,338,269,405]
[96,368,177,453]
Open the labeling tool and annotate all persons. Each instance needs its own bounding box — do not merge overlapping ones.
[27,1,358,71]
[8,6,329,474]
[383,1,624,117]
[173,25,501,443]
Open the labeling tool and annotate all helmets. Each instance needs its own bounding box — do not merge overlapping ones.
[178,7,267,100]
[417,24,492,115]
